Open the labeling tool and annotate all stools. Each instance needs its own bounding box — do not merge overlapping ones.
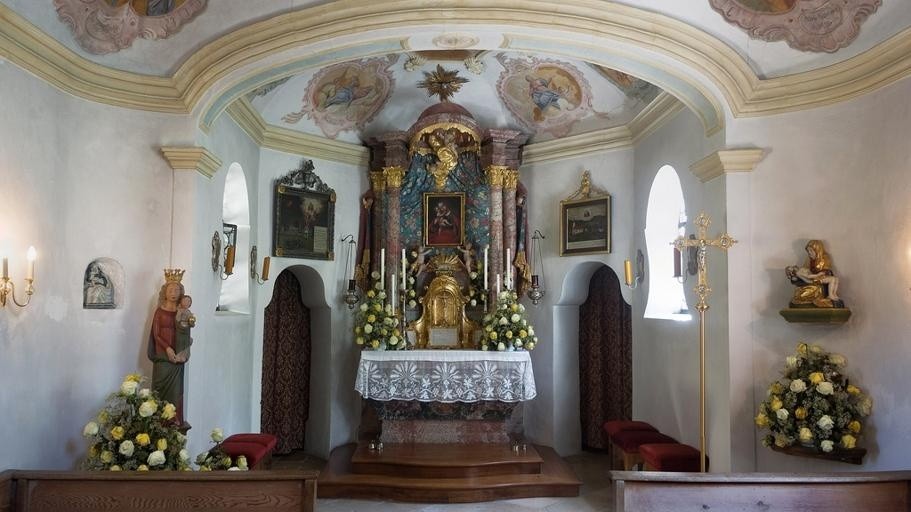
[611,430,677,471]
[605,420,658,484]
[222,443,267,471]
[639,444,708,473]
[225,434,277,470]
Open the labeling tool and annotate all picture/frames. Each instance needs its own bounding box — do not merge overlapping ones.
[272,160,335,260]
[560,171,611,256]
[423,191,465,248]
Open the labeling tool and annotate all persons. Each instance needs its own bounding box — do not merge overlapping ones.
[433,201,456,235]
[318,77,374,110]
[792,239,832,304]
[148,281,194,429]
[174,294,194,357]
[526,72,565,114]
[786,263,843,303]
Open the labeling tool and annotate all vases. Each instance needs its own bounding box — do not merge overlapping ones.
[378,341,387,352]
[507,341,514,351]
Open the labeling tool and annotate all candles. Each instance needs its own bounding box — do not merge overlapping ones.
[226,247,235,272]
[625,259,632,283]
[3,256,8,279]
[349,279,355,290]
[484,248,511,300]
[381,248,406,314]
[28,246,35,278]
[262,256,270,278]
[532,275,538,286]
[674,248,681,276]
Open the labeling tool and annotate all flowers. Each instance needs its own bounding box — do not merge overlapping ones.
[755,341,873,455]
[354,291,400,349]
[481,290,538,351]
[80,372,248,471]
